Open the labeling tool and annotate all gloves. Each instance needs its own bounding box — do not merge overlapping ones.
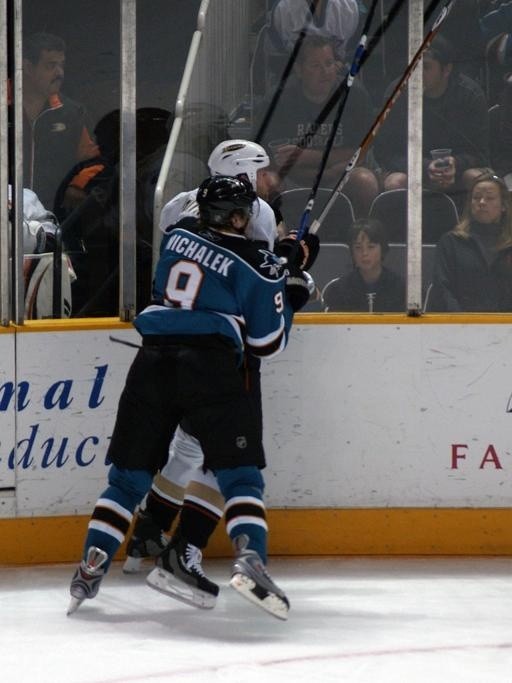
[279,228,320,312]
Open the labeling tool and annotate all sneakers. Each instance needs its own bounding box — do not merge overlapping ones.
[126,529,170,557]
[69,560,105,598]
[154,541,218,596]
[230,550,289,611]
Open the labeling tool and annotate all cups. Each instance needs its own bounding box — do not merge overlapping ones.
[430,148,452,177]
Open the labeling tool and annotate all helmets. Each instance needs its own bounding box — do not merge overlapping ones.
[208,139,271,191]
[197,176,257,229]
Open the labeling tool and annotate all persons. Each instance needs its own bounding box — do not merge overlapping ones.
[111,136,323,602]
[67,173,303,611]
[5,1,510,314]
